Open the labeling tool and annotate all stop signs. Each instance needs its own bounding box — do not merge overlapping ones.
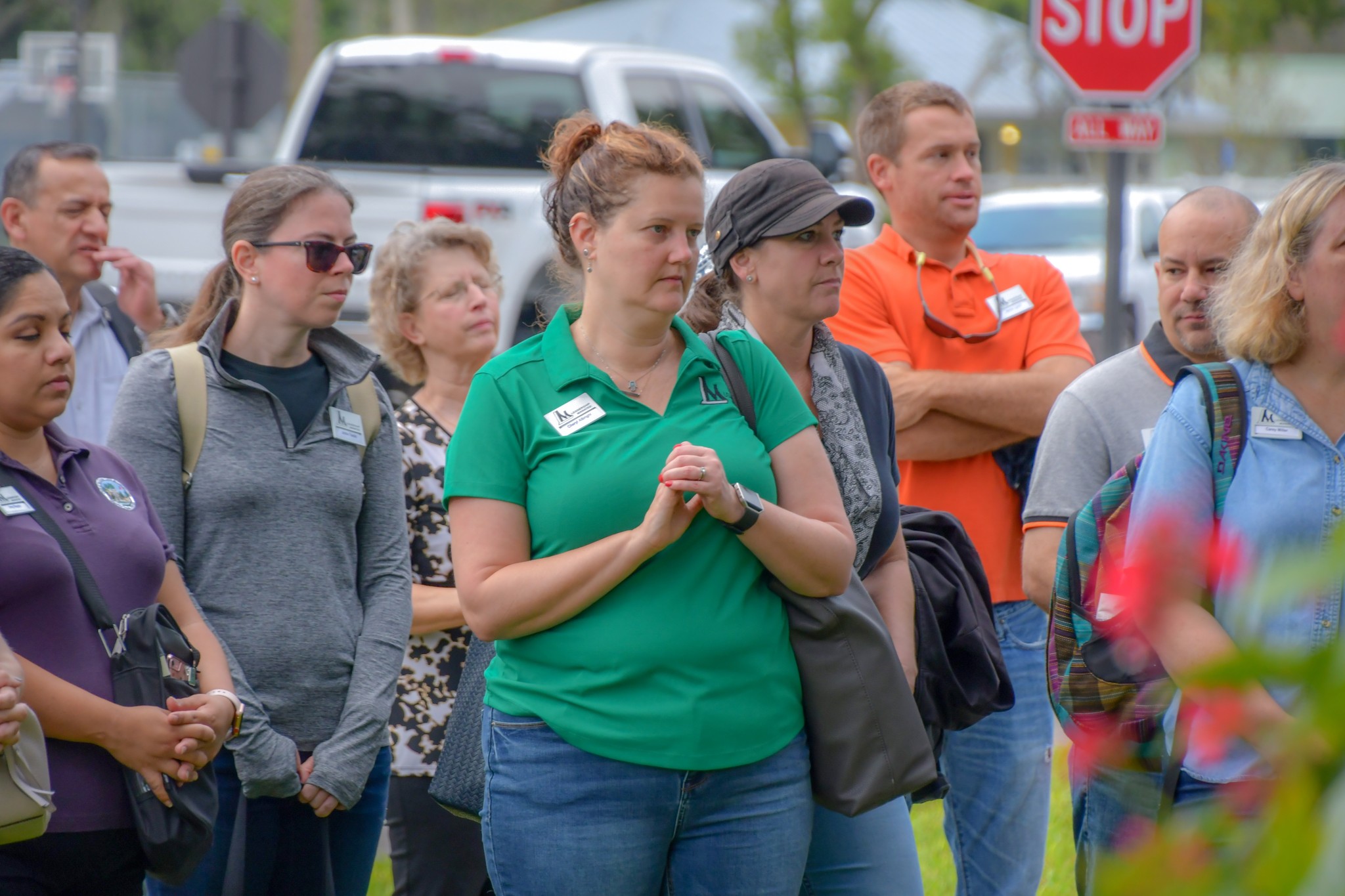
[1031,0,1202,106]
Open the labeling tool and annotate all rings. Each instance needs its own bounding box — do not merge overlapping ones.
[698,466,706,481]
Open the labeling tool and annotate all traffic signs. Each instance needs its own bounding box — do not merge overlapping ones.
[1062,107,1167,152]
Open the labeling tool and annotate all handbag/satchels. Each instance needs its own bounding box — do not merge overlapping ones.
[426,627,497,825]
[762,563,939,819]
[0,703,57,845]
[113,600,220,884]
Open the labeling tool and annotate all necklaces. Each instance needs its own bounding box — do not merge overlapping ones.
[574,318,672,397]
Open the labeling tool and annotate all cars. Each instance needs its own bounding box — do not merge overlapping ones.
[963,187,1187,365]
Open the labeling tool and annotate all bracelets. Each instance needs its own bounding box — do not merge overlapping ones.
[132,302,181,344]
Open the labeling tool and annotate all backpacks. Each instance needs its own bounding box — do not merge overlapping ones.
[1042,362,1249,779]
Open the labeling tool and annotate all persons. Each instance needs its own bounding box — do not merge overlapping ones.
[443,112,856,896]
[107,164,411,896]
[678,156,929,896]
[0,634,27,756]
[1,244,245,895]
[1,140,182,451]
[146,256,243,352]
[1022,183,1267,896]
[1119,161,1344,896]
[821,80,1096,894]
[364,215,499,896]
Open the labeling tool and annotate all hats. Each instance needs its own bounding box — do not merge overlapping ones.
[704,157,875,280]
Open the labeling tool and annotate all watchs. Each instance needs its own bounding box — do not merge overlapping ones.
[203,688,245,745]
[723,482,764,536]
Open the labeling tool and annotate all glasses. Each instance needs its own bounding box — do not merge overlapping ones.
[252,240,373,274]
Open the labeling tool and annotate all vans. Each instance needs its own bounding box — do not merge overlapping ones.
[270,33,849,371]
